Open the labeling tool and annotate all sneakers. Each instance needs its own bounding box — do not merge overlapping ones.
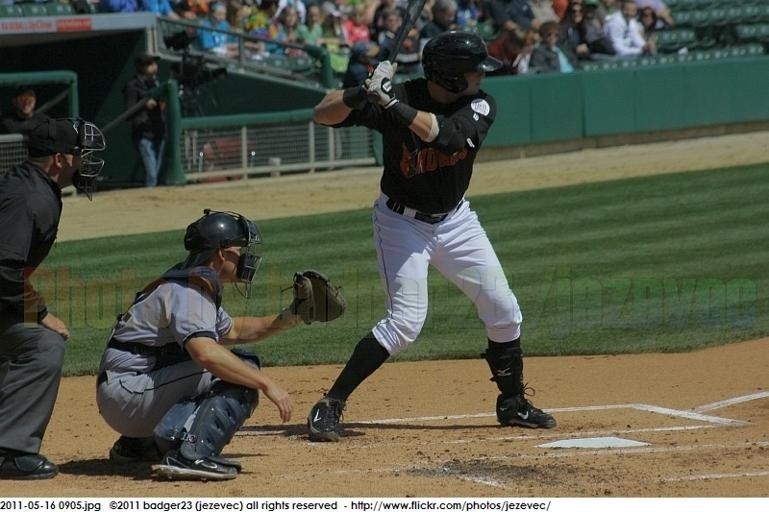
[0,455,58,480]
[496,386,556,428]
[109,434,155,464]
[151,447,238,480]
[307,391,346,442]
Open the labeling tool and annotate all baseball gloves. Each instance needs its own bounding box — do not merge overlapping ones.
[292,271,346,325]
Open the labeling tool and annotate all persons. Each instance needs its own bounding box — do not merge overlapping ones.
[125,56,170,186]
[306,31,557,440]
[97,208,347,483]
[4,88,47,137]
[109,0,672,95]
[1,116,108,481]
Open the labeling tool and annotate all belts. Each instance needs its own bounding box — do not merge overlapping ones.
[386,198,464,224]
[97,369,142,386]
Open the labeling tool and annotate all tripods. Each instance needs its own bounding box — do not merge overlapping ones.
[129,97,231,186]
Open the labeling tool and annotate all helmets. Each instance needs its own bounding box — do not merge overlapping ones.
[26,114,107,202]
[422,30,503,92]
[184,209,263,282]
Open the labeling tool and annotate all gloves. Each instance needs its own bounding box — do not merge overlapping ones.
[365,77,399,110]
[371,60,398,81]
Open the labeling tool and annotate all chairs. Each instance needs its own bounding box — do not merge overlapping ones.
[0,4,75,16]
[245,54,329,82]
[581,0,769,69]
[462,19,499,41]
[161,19,203,53]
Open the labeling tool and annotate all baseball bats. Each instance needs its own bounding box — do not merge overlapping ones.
[367,0,428,105]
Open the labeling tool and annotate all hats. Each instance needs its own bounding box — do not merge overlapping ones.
[135,55,160,67]
